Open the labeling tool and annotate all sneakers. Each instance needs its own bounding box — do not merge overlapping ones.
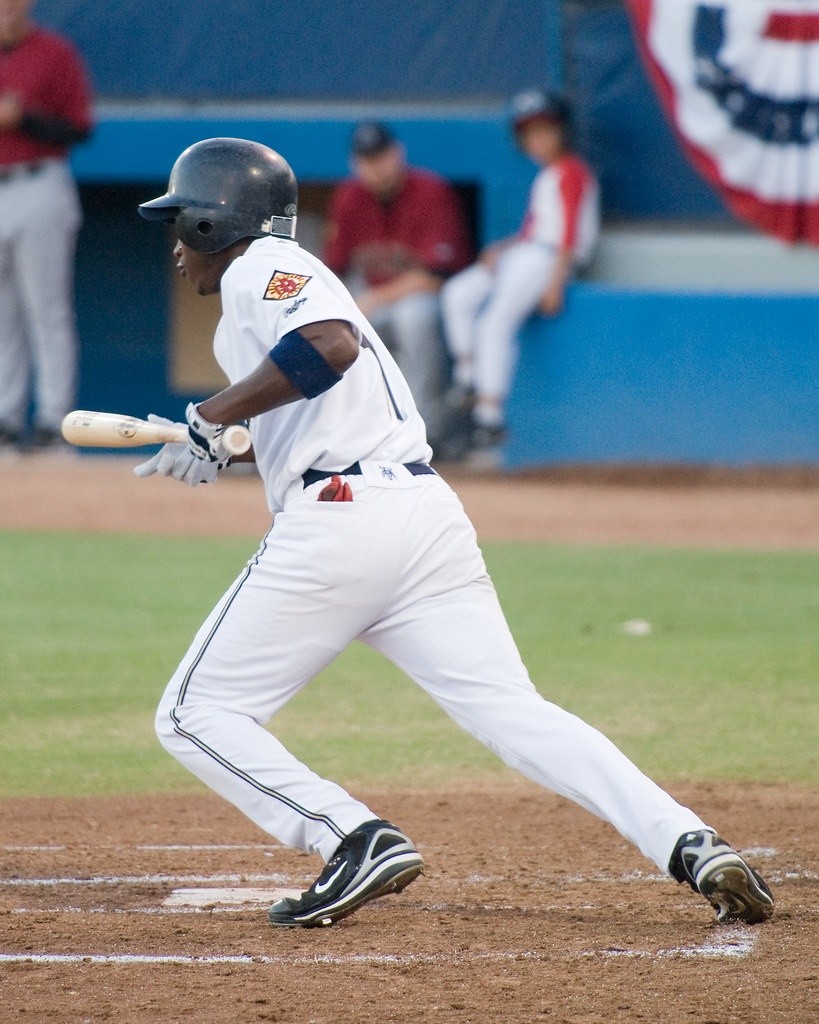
[268,819,425,928]
[678,830,774,923]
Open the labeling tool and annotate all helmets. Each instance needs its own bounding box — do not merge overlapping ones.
[512,90,578,152]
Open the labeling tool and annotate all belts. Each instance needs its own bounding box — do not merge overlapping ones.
[303,459,436,488]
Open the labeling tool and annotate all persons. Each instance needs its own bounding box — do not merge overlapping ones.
[324,120,474,447]
[440,90,602,444]
[156,138,775,926]
[1,0,98,449]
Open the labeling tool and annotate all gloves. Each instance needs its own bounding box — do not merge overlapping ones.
[185,401,226,463]
[134,413,233,488]
[317,475,353,502]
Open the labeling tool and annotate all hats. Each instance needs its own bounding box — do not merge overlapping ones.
[352,122,390,157]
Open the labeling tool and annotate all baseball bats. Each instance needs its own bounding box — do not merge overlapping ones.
[59,408,252,458]
